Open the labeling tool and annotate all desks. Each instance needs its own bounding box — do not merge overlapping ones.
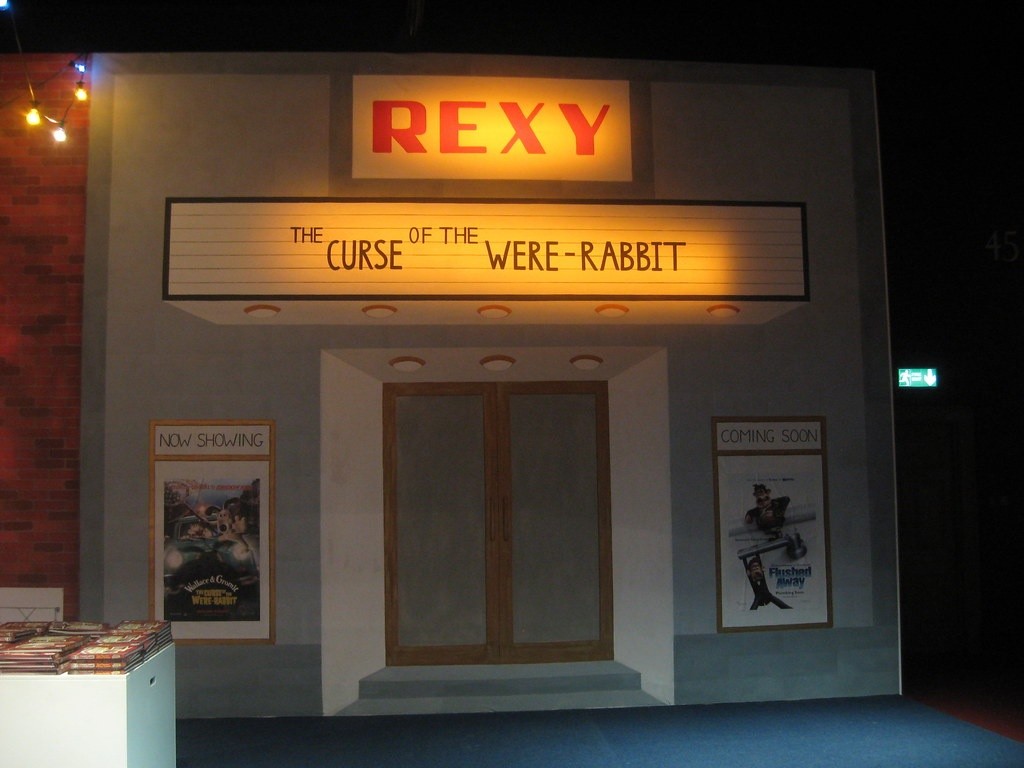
[0,643,176,768]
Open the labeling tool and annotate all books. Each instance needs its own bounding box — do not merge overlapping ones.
[0,619,173,673]
